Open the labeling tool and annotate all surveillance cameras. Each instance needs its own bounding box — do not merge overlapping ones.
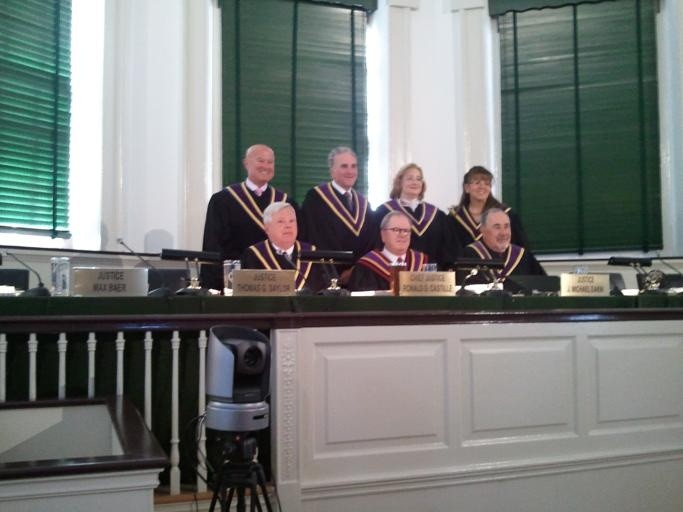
[204,324,274,425]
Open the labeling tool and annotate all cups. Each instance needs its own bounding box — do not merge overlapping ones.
[223,259,242,297]
[574,265,588,276]
[392,262,408,297]
[423,263,436,271]
[50,256,71,298]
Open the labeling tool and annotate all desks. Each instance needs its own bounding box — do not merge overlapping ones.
[0,296,683,312]
[0,397,170,512]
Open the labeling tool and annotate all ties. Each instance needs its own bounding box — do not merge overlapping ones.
[397,256,402,264]
[344,190,356,212]
[256,188,263,196]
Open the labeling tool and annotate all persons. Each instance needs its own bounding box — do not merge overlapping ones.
[302,147,378,288]
[242,202,331,292]
[445,167,528,269]
[374,164,448,271]
[452,207,548,290]
[348,212,439,290]
[201,144,316,289]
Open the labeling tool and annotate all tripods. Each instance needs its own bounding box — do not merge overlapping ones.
[208,434,274,512]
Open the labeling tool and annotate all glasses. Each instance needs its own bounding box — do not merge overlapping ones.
[381,226,412,233]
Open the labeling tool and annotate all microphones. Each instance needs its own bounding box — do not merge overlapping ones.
[282,253,313,293]
[8,252,51,296]
[456,267,477,297]
[117,239,175,296]
